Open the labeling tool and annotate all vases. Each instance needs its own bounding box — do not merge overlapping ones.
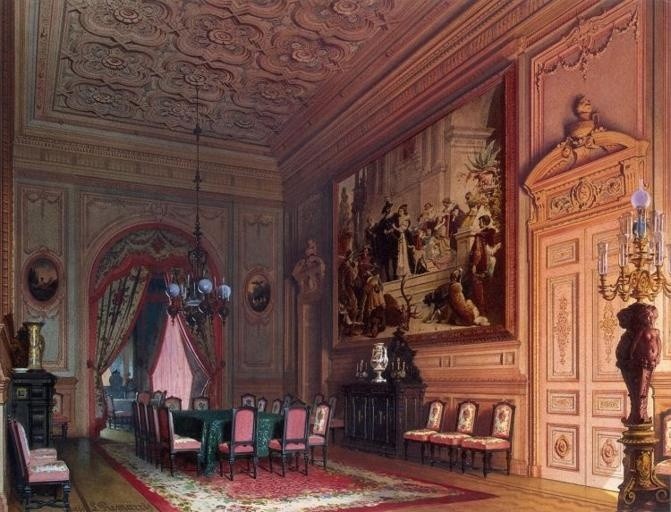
[368,341,390,384]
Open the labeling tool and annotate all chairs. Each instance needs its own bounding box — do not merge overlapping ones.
[404,395,447,465]
[103,388,336,478]
[6,417,61,503]
[428,398,477,470]
[649,406,671,483]
[13,419,72,512]
[460,399,514,477]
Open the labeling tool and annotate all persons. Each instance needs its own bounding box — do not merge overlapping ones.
[612,307,635,424]
[334,190,502,338]
[628,306,663,422]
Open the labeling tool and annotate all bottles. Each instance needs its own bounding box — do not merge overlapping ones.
[371,342,389,383]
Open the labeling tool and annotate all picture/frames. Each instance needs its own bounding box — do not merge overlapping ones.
[326,59,519,355]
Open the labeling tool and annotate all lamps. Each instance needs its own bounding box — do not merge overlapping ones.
[162,84,230,328]
[594,174,670,512]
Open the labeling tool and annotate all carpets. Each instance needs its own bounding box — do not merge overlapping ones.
[96,435,500,512]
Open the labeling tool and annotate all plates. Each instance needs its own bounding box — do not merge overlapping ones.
[12,367,30,373]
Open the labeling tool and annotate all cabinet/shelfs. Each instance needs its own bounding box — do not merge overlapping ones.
[337,383,425,459]
[9,370,53,448]
